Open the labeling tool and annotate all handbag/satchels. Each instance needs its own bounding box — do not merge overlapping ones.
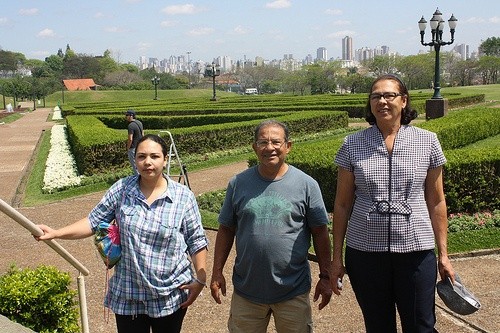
[94,221,122,269]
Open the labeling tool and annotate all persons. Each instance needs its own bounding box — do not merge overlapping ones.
[30,134,208,333]
[330,75,454,333]
[125,110,144,175]
[210,121,332,333]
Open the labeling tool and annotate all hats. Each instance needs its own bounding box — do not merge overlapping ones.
[437,272,482,315]
[125,110,135,117]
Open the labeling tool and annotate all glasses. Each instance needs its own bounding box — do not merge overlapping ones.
[255,139,286,149]
[368,91,402,101]
[126,114,129,116]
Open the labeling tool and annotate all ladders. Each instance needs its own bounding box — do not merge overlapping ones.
[143,130,192,189]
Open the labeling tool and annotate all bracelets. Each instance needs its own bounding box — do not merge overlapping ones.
[195,278,207,288]
[319,274,329,278]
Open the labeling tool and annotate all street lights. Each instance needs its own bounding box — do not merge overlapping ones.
[150,74,161,101]
[206,61,220,100]
[419,7,459,120]
